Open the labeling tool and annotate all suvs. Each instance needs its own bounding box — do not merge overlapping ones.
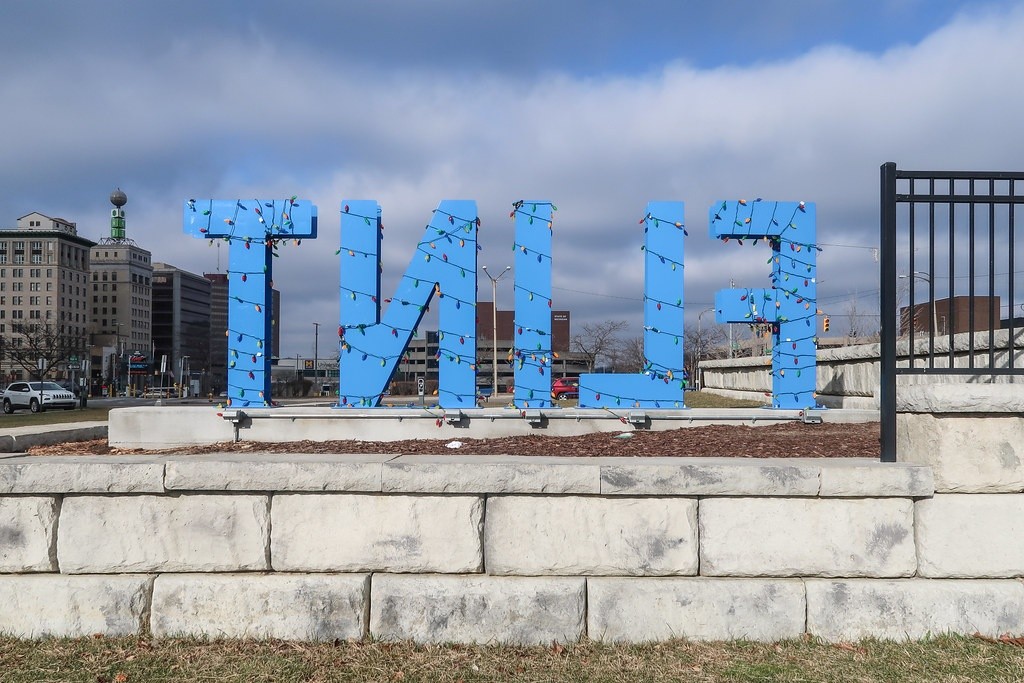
[550,377,578,401]
[2,380,77,414]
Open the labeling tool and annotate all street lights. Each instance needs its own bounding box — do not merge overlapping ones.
[312,322,322,385]
[178,356,190,399]
[483,265,512,397]
[900,271,938,337]
[114,322,125,391]
[698,308,717,392]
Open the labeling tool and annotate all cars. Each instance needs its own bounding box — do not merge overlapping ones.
[146,390,167,398]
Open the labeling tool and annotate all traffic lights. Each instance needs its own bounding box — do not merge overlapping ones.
[823,317,830,333]
